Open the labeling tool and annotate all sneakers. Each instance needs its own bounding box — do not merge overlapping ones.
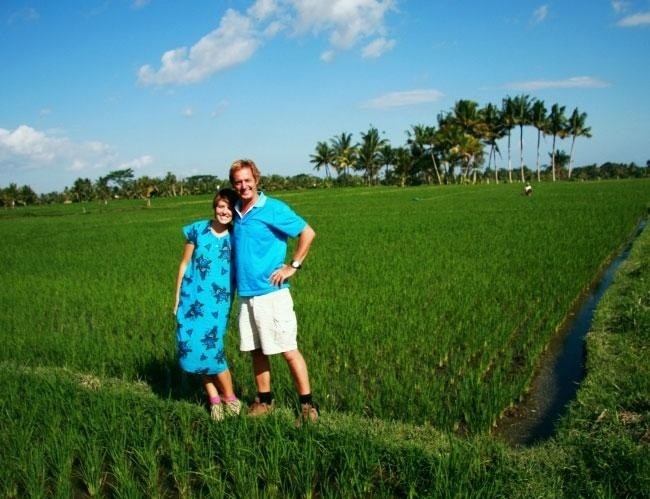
[209,403,224,421]
[296,403,318,426]
[224,396,242,417]
[247,397,274,416]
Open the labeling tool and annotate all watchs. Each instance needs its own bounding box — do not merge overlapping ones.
[290,259,302,269]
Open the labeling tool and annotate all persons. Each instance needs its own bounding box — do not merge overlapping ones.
[229,160,319,428]
[524,182,532,197]
[173,188,241,422]
